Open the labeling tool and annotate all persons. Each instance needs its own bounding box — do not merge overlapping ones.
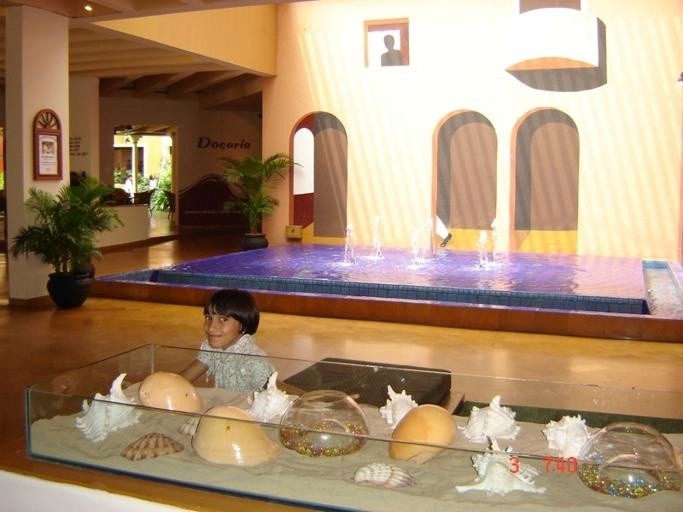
[123,175,135,205]
[379,35,404,66]
[148,175,156,190]
[174,287,277,392]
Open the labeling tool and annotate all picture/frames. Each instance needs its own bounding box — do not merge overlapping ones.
[33,109,63,180]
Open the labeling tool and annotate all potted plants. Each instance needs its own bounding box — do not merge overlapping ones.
[218,153,302,251]
[9,175,125,310]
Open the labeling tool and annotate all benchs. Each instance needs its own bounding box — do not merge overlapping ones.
[280,358,465,414]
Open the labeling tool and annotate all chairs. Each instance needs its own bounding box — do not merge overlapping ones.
[163,190,175,220]
[114,189,156,215]
